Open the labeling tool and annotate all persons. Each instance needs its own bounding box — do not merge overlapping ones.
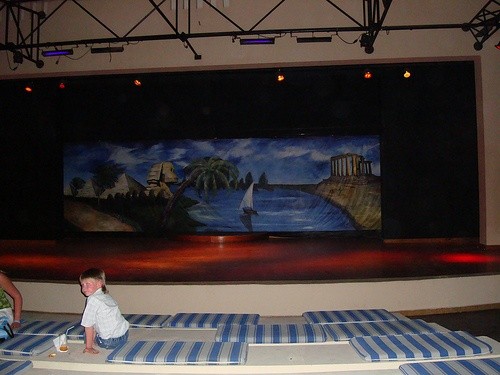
[0,269,23,339]
[79,267,130,354]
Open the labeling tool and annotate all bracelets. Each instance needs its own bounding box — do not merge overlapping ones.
[14,320,21,324]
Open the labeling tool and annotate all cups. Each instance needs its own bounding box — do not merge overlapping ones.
[59,333,67,351]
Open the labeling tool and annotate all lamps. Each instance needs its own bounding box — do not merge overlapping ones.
[240,38,275,45]
[296,37,331,43]
[91,45,124,53]
[41,49,73,57]
